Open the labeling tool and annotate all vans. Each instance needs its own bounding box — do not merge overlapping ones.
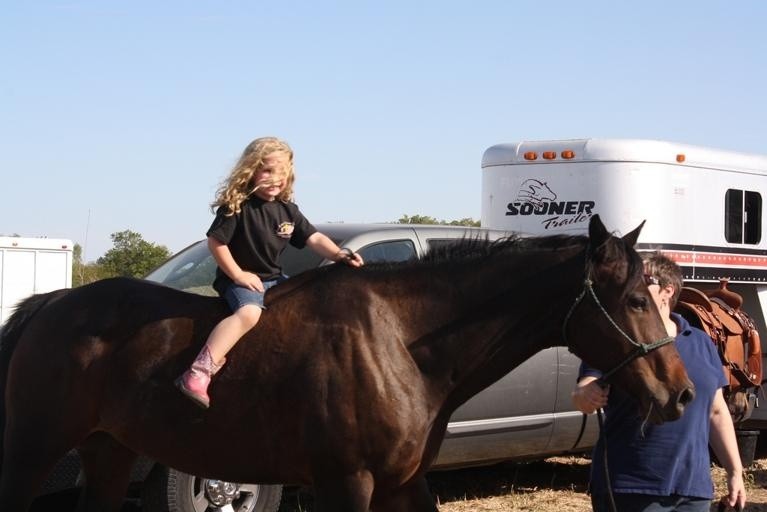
[133,220,605,512]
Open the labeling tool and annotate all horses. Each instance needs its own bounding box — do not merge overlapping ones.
[0,214,695,512]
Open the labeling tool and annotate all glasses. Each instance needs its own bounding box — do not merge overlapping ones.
[644,274,660,286]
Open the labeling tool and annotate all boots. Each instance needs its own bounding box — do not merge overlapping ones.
[174,344,227,409]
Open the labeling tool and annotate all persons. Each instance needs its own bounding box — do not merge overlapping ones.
[572,250,747,512]
[172,136,364,411]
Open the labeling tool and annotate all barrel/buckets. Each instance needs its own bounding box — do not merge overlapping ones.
[734,429,760,468]
